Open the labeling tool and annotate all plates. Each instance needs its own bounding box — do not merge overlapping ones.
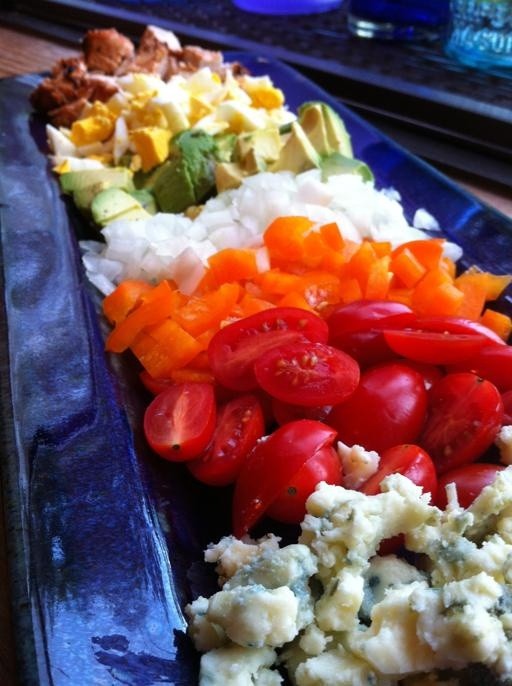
[0,49,511,685]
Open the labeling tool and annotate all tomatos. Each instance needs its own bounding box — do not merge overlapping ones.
[142,301,512,556]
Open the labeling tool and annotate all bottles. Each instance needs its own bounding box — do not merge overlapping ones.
[234,0,510,68]
